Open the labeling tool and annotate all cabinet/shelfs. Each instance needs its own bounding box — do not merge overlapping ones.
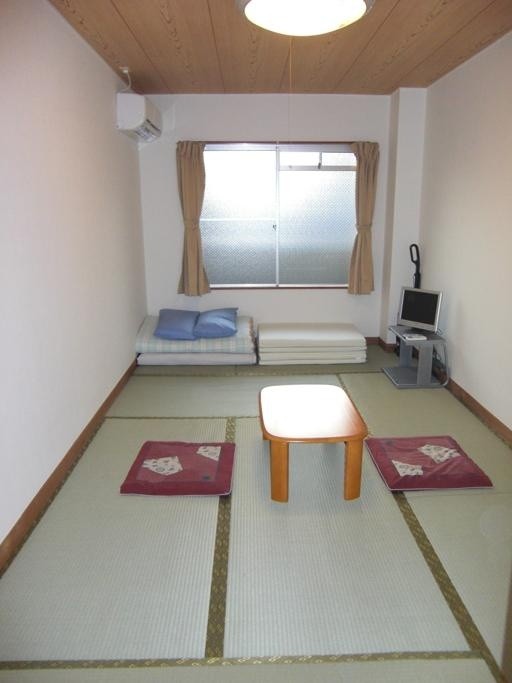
[381,326,452,390]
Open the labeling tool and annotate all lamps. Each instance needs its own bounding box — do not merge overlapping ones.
[237,0,373,38]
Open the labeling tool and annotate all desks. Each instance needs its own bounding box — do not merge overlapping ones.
[260,384,368,502]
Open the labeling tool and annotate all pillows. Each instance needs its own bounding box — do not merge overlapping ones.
[192,307,237,339]
[155,309,199,341]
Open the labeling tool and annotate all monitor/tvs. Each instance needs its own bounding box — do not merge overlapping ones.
[397,287,443,332]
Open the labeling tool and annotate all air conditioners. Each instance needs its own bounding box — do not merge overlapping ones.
[114,91,163,145]
